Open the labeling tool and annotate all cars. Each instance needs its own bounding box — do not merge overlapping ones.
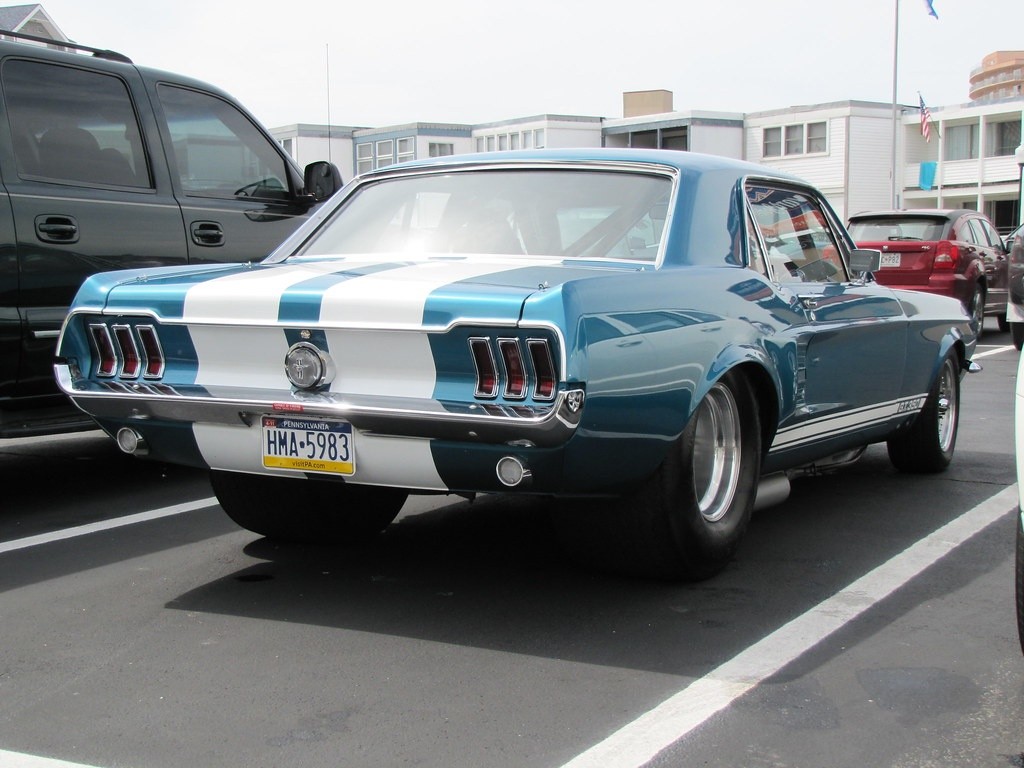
[47,144,985,574]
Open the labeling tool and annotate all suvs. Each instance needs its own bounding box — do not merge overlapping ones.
[823,207,1011,339]
[0,28,348,439]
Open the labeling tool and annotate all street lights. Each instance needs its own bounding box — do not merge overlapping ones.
[1014,144,1024,228]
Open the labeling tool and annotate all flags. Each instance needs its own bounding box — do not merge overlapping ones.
[918,161,936,192]
[921,0,940,21]
[919,95,932,144]
